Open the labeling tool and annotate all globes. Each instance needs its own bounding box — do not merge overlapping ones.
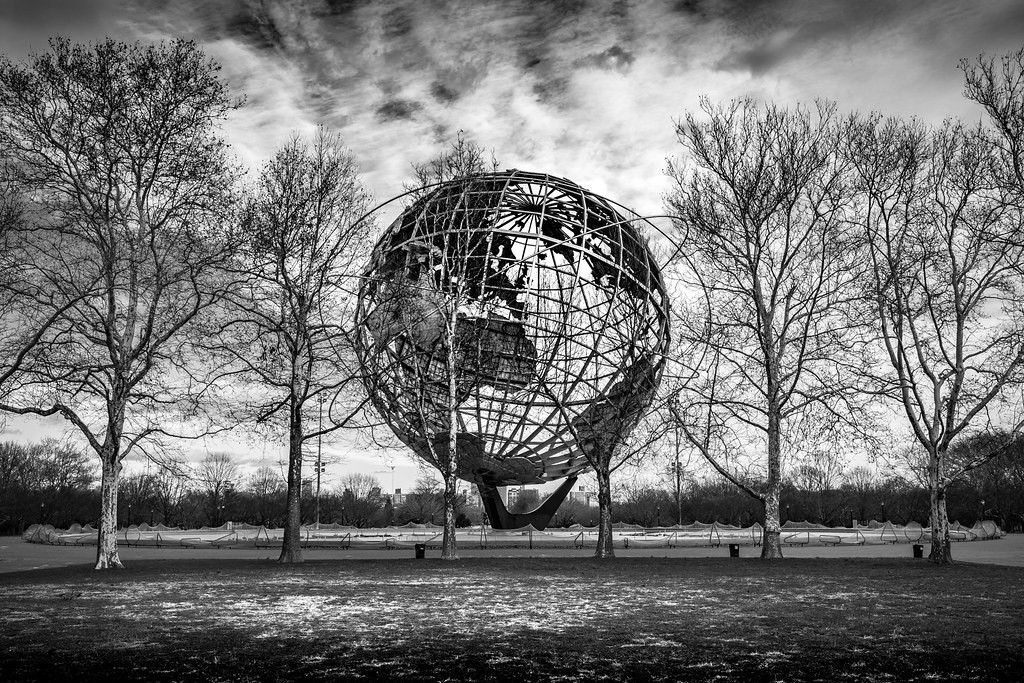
[349,169,673,487]
